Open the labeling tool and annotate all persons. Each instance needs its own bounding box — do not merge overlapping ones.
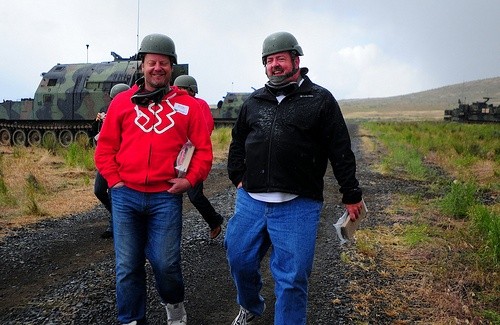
[95,83,131,238]
[94,34,213,325]
[224,32,363,325]
[173,75,226,239]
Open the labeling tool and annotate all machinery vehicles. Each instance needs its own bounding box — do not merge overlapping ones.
[443,97,500,124]
[0,52,188,149]
[208,86,256,129]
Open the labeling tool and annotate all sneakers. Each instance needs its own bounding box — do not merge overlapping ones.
[231,302,266,325]
[166,302,187,325]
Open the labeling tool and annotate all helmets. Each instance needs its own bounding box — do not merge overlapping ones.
[138,34,177,65]
[174,75,198,94]
[262,32,304,61]
[110,83,130,98]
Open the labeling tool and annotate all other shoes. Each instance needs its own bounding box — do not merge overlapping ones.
[101,227,113,239]
[211,217,223,239]
